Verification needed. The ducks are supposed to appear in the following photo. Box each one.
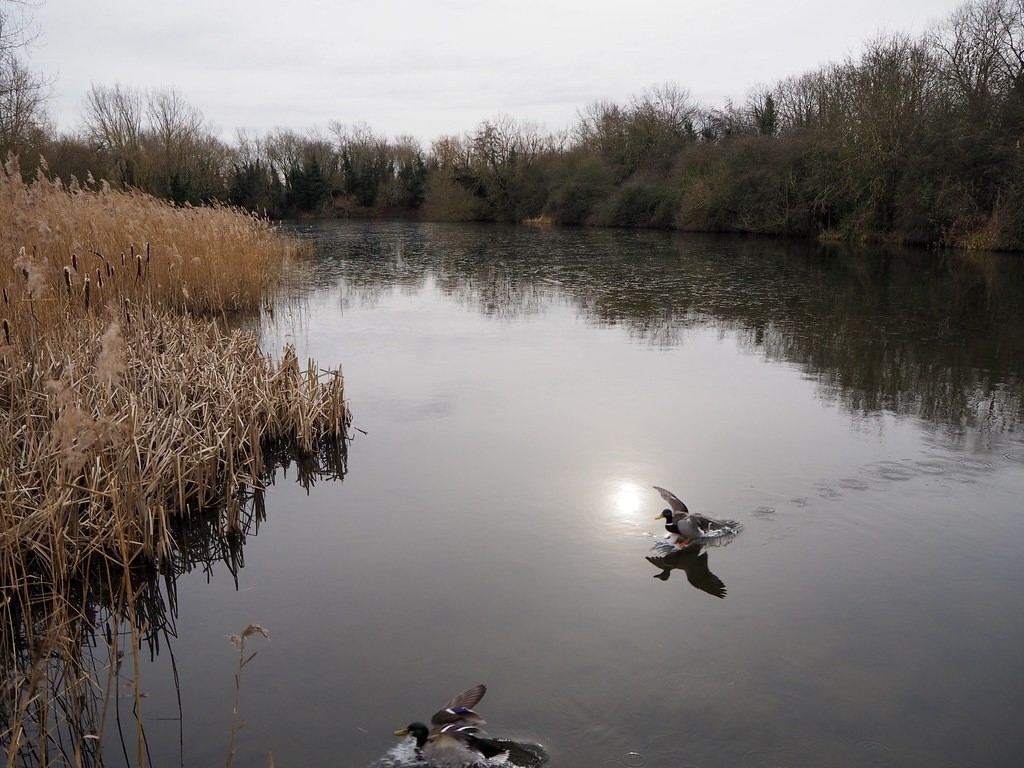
[653,486,724,548]
[393,684,549,768]
